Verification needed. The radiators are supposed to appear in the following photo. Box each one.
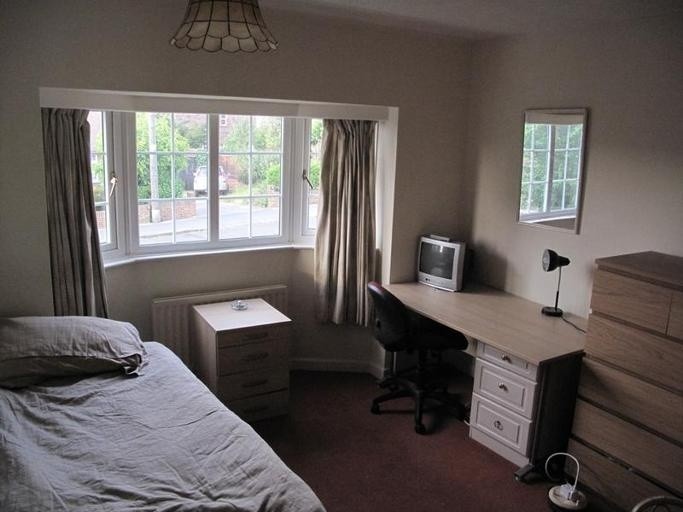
[151,284,289,367]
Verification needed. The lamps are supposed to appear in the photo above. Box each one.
[541,249,570,316]
[169,0,280,56]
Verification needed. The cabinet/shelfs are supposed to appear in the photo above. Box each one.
[561,250,683,512]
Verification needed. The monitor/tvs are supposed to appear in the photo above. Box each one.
[416,234,475,292]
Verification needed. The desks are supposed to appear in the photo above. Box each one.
[382,280,588,480]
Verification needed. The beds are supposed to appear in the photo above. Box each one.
[0,342,327,512]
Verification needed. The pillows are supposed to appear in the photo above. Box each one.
[0,315,146,390]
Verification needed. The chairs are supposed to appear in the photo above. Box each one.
[367,281,467,433]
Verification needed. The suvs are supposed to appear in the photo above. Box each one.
[193,164,230,197]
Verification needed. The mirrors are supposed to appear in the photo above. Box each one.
[512,106,588,236]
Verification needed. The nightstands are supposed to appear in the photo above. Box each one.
[193,297,295,426]
